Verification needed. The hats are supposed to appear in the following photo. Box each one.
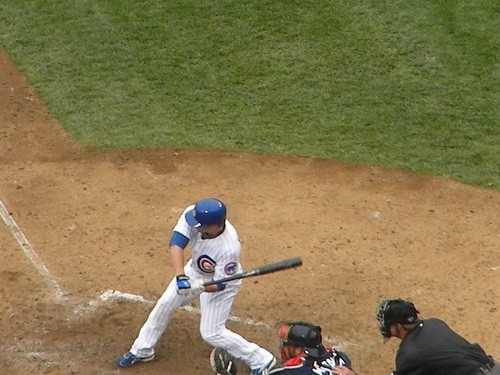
[185,199,227,228]
[377,299,418,344]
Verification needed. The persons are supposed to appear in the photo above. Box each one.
[331,299,500,374]
[115,198,277,375]
[266,322,351,374]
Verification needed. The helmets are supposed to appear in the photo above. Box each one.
[277,320,332,362]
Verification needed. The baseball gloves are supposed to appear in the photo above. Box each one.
[207,348,239,375]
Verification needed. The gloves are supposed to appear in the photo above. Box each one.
[191,280,204,296]
[177,275,191,296]
[210,347,238,375]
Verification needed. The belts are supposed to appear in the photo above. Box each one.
[470,355,495,375]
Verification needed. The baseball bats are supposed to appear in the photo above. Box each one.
[176,256,303,296]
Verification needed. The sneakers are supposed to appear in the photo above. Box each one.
[115,352,155,367]
[250,357,276,375]
